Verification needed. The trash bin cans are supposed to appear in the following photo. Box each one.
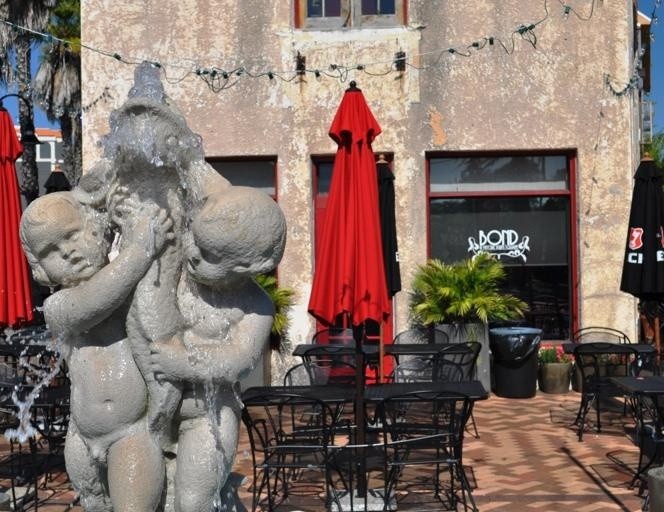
[489,326,543,398]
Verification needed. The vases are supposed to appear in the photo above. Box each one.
[538,363,570,394]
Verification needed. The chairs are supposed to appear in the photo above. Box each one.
[572,327,664,510]
[0,317,78,512]
[232,326,481,512]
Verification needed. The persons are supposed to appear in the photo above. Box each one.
[19,192,167,512]
[111,182,287,512]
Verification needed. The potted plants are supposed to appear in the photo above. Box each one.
[237,272,297,392]
[407,252,530,392]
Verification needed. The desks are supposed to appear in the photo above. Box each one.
[561,343,656,433]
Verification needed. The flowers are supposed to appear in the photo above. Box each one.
[535,338,576,375]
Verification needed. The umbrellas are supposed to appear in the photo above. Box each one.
[308,81,390,499]
[619,152,664,366]
[0,107,35,326]
